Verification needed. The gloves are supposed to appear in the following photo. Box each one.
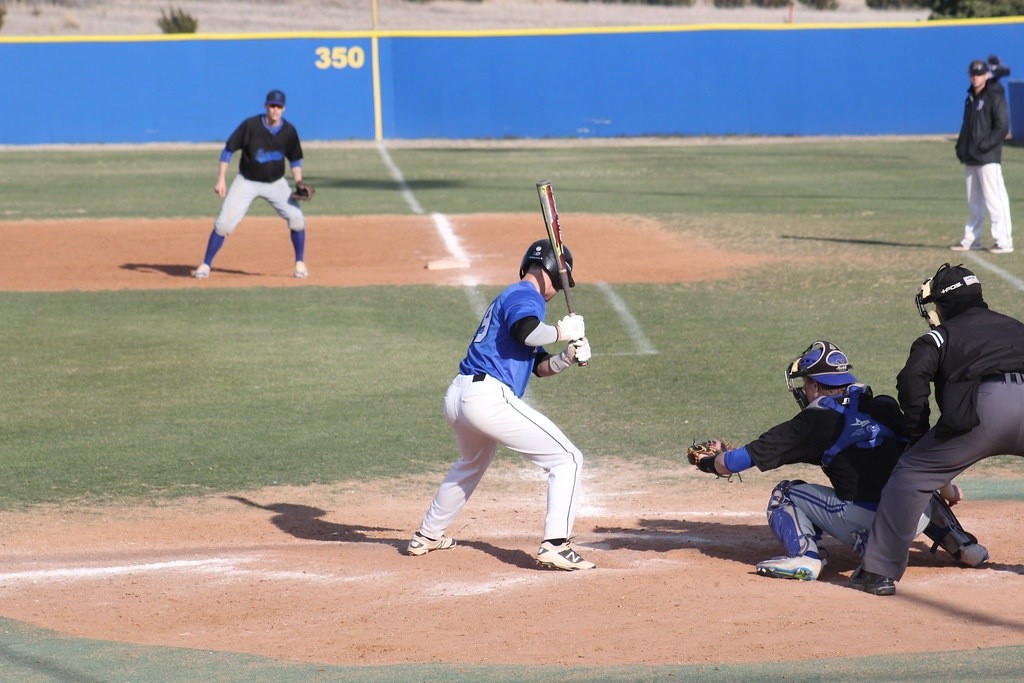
[557,314,585,341]
[565,337,592,363]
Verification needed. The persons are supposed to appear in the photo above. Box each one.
[195,89,314,279]
[849,264,1024,595]
[693,339,988,581]
[949,60,1015,254]
[407,238,597,570]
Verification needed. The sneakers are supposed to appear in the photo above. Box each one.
[406,531,457,556]
[960,543,988,568]
[534,536,596,570]
[756,554,827,581]
[848,572,895,595]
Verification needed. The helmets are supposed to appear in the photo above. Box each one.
[799,347,857,386]
[520,239,575,287]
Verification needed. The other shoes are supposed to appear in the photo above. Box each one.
[988,245,1014,253]
[195,263,210,278]
[951,242,982,250]
[295,261,307,278]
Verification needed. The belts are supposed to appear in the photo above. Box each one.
[472,374,485,382]
[853,499,879,512]
[981,374,1024,382]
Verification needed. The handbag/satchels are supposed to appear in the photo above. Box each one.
[935,378,981,440]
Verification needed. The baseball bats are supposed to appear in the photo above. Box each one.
[536,179,590,366]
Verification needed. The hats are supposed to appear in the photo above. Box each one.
[969,61,989,74]
[267,90,285,107]
[931,266,982,302]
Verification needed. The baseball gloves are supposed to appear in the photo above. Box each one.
[688,437,738,475]
[290,182,316,201]
